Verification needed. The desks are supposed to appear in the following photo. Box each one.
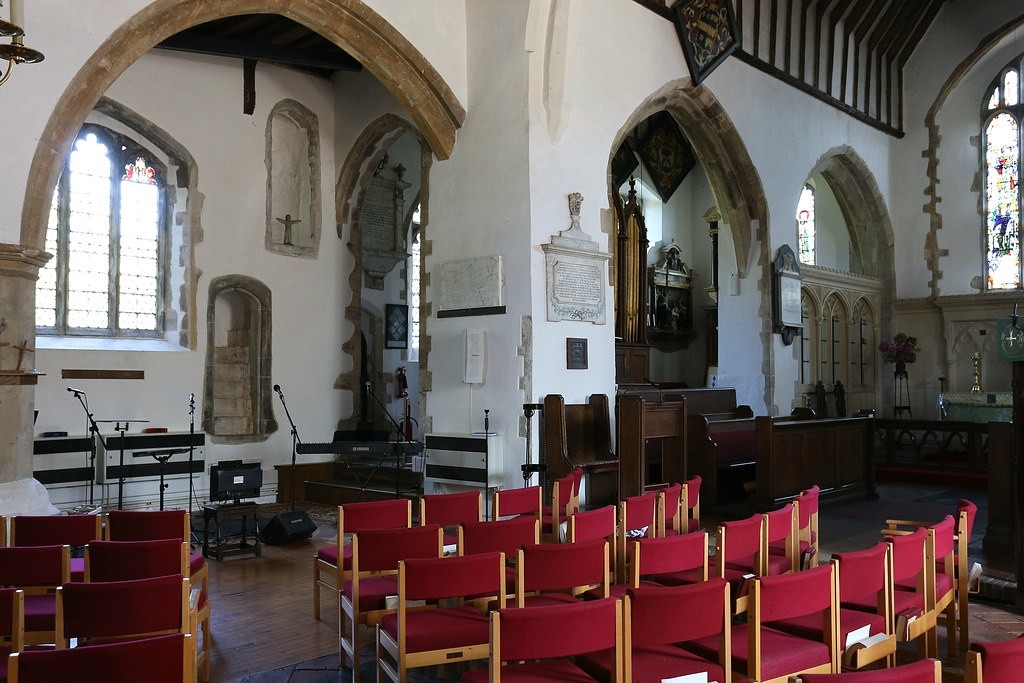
[939,392,1013,407]
[201,502,263,562]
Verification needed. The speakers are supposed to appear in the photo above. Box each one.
[261,511,317,545]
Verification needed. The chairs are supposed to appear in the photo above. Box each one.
[0,510,210,683]
[313,465,1024,683]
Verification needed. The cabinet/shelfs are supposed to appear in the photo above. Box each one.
[424,433,503,489]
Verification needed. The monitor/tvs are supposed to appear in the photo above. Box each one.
[210,463,263,508]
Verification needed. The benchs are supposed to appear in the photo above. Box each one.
[689,406,816,511]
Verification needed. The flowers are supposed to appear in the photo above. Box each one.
[878,332,919,365]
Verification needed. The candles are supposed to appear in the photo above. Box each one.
[9,0,25,35]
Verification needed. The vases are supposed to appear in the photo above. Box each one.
[895,362,906,374]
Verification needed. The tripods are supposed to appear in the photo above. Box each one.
[189,402,215,544]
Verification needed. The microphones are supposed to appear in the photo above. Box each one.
[366,381,371,397]
[643,377,662,402]
[189,392,194,402]
[66,387,85,394]
[273,384,284,396]
